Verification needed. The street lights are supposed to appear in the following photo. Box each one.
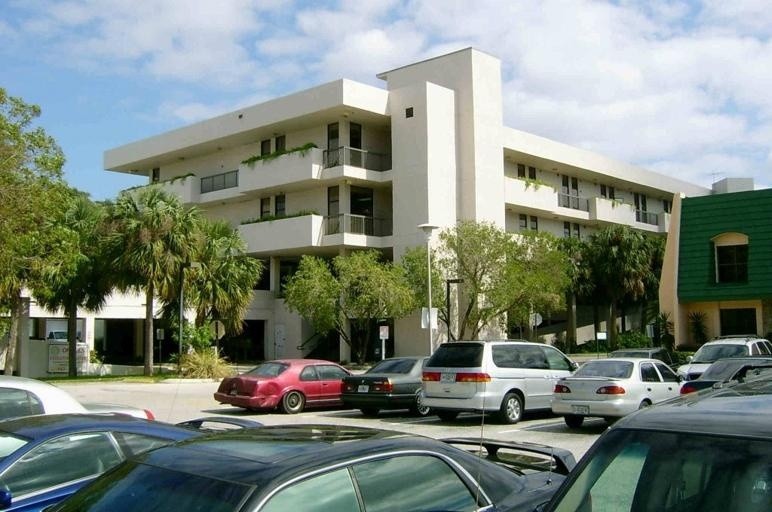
[418,223,440,356]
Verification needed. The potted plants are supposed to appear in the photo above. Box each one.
[656,313,674,349]
